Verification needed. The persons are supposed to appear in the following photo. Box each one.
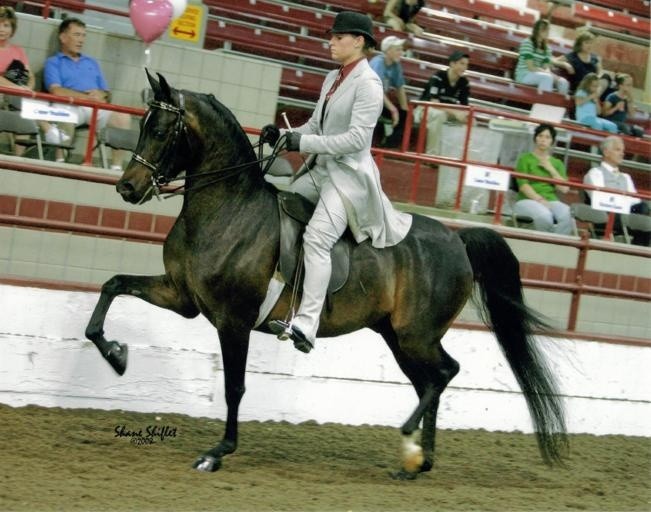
[603,73,643,137]
[43,18,132,171]
[383,0,425,37]
[512,124,579,236]
[582,135,651,247]
[515,19,569,95]
[259,11,413,353]
[0,7,70,156]
[553,32,612,97]
[412,50,470,124]
[368,35,409,150]
[574,72,618,134]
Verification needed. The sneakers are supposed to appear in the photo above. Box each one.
[44,123,60,147]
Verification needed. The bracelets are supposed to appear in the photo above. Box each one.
[537,198,544,202]
[391,108,398,114]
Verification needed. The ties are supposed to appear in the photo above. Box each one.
[324,67,343,101]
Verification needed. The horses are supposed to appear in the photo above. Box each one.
[85,66,575,483]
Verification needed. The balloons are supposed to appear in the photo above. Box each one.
[128,0,173,44]
[172,0,187,20]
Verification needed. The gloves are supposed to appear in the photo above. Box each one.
[285,130,301,151]
[260,124,280,147]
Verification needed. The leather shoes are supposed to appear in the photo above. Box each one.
[267,319,311,354]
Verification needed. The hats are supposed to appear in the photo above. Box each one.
[325,10,377,47]
[380,35,406,51]
[448,50,470,61]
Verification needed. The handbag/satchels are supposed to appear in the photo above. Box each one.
[2,59,29,86]
